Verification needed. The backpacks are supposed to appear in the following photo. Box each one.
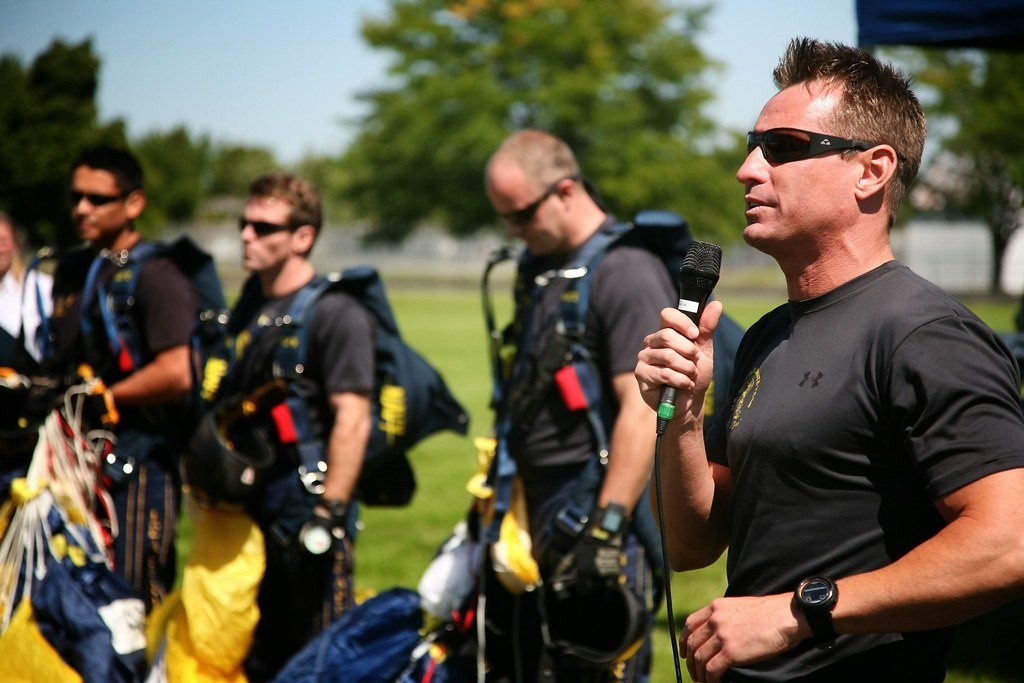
[110,237,228,350]
[286,266,469,455]
[569,212,755,462]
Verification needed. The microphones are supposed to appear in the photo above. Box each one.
[656,241,722,435]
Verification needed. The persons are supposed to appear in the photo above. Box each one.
[481,37,1024,683]
[1,143,380,683]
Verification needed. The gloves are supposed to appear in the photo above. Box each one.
[296,497,355,575]
[552,502,630,592]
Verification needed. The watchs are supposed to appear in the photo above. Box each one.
[794,575,838,651]
[319,497,347,517]
[590,502,634,535]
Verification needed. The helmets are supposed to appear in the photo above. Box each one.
[547,546,655,665]
[185,410,273,515]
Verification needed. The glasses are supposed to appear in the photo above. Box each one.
[505,176,578,225]
[747,128,906,163]
[238,216,298,237]
[69,192,129,205]
